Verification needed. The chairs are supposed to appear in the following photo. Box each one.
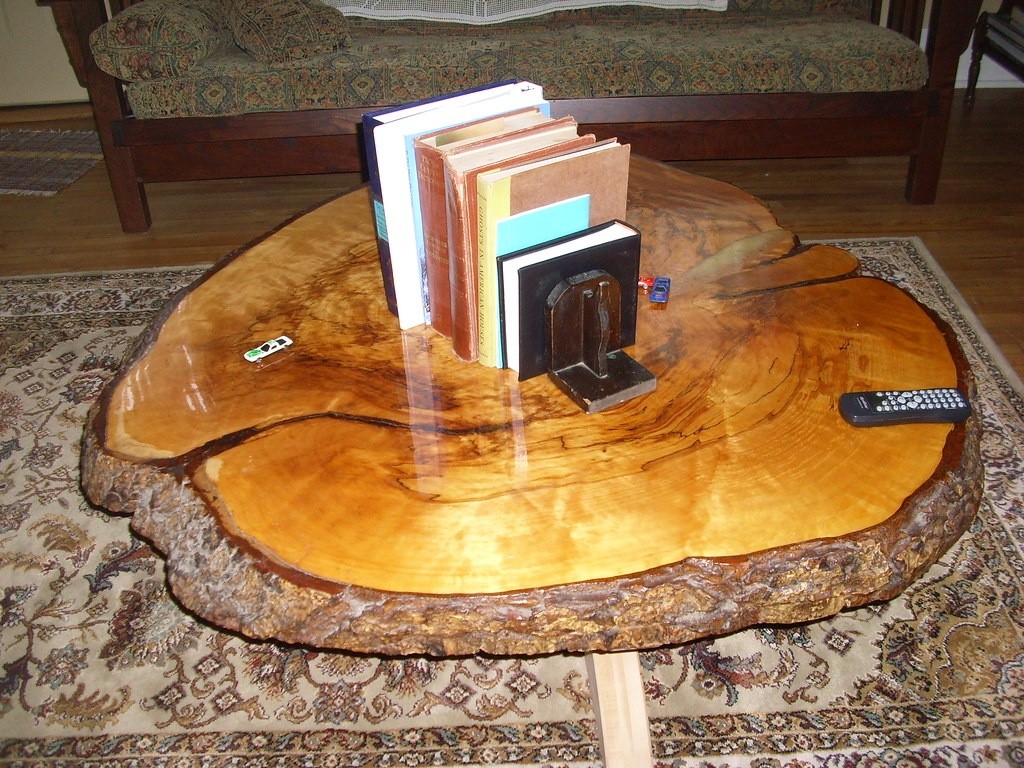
[962,0,1024,116]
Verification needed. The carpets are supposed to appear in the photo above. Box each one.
[0,126,104,198]
[0,235,1024,767]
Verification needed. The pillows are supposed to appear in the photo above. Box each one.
[220,0,354,64]
[88,0,237,84]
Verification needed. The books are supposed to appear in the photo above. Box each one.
[360,78,642,381]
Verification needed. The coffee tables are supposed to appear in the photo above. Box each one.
[81,149,986,658]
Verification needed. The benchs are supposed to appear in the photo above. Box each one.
[36,0,982,236]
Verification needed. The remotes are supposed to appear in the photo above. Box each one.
[837,388,972,427]
